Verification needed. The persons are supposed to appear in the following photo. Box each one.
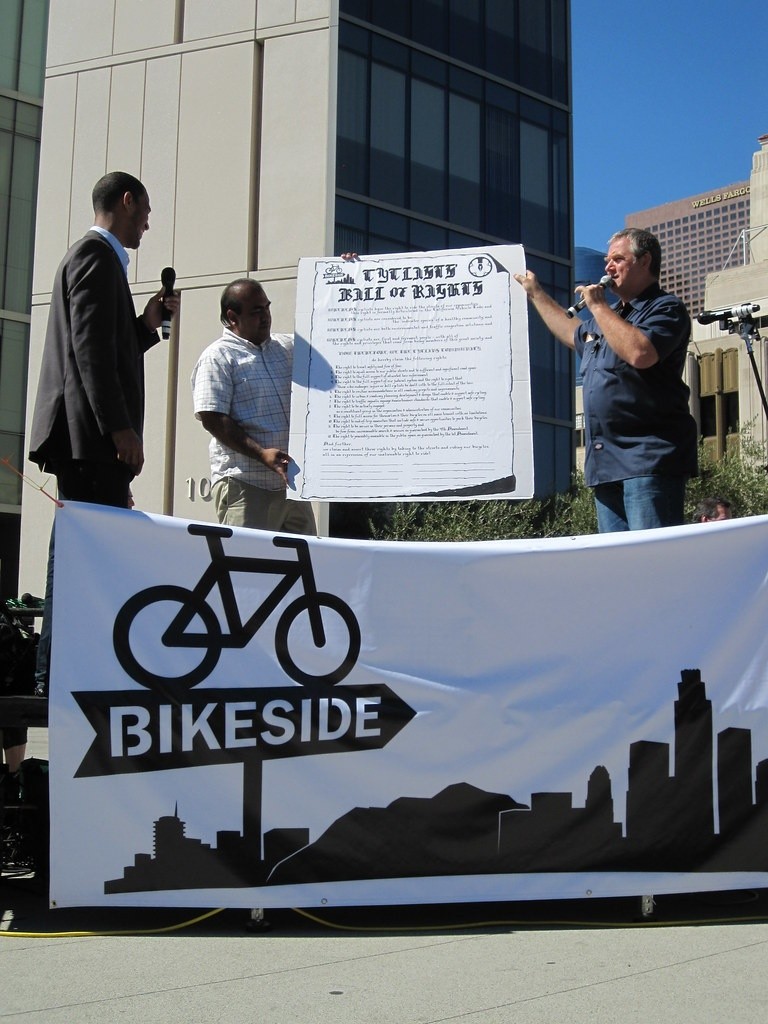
[691,494,733,522]
[29,169,178,695]
[193,279,321,535]
[515,225,698,532]
[0,606,33,793]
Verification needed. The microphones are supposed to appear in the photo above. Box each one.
[161,267,176,339]
[697,304,760,325]
[565,275,614,318]
